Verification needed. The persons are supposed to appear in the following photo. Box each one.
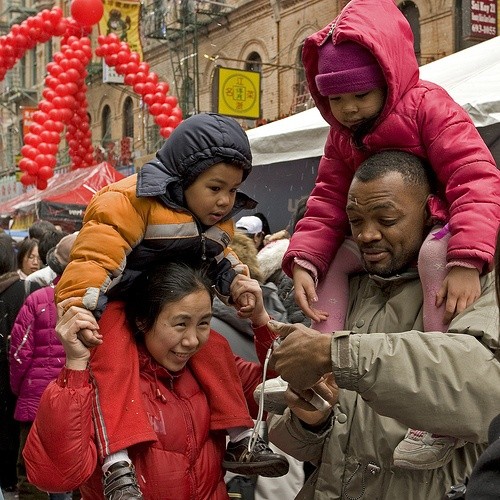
[464,437,500,500]
[56,114,288,500]
[15,195,312,361]
[266,154,499,500]
[252,0,500,469]
[10,230,81,500]
[0,232,26,491]
[21,258,279,500]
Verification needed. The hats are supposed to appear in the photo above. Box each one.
[315,34,386,95]
[46,231,80,274]
[236,216,264,234]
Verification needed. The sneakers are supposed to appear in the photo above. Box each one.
[253,376,289,415]
[104,461,144,500]
[393,428,468,471]
[222,434,289,477]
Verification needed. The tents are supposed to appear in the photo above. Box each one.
[0,161,127,232]
[233,36,499,173]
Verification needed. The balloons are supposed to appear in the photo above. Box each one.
[0,1,186,190]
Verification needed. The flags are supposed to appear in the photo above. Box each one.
[97,2,144,84]
